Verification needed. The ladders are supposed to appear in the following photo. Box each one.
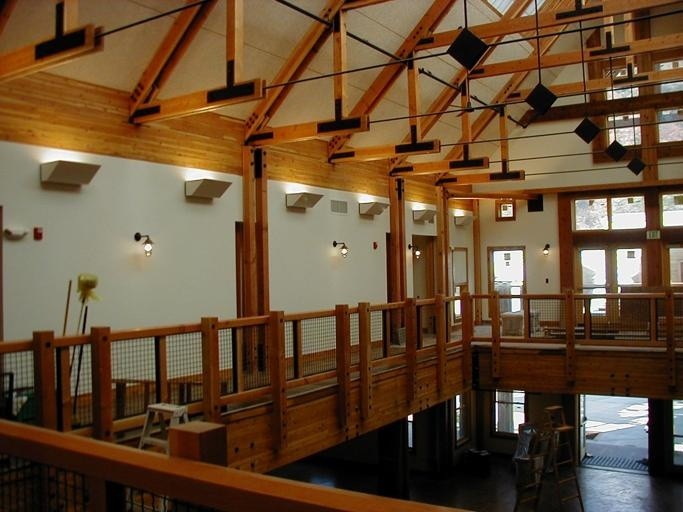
[123,403,190,512]
[513,405,584,512]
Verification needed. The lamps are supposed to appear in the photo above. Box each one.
[408,243,422,261]
[358,201,389,218]
[542,243,551,256]
[333,241,351,258]
[132,231,155,257]
[284,192,323,211]
[413,207,476,226]
[38,157,102,190]
[185,178,232,202]
